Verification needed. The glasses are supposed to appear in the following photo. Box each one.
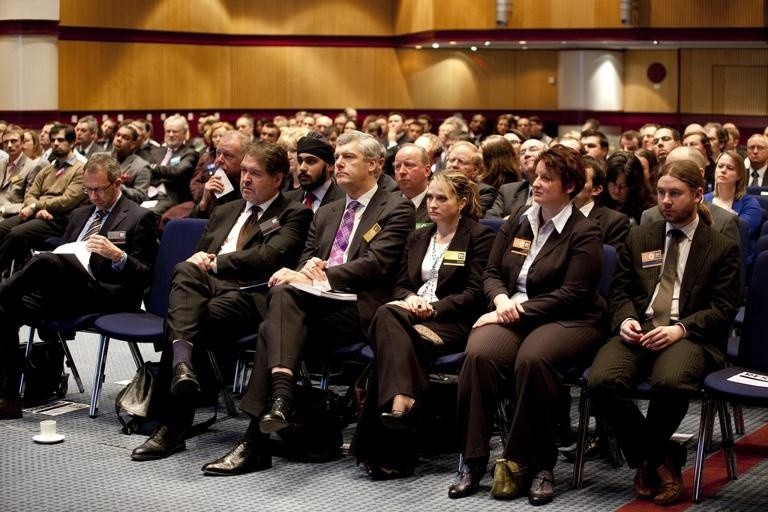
[82,183,112,194]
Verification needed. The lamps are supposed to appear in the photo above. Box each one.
[617,2,641,26]
[493,0,514,27]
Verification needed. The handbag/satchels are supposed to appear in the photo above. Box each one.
[605,429,625,467]
[350,385,420,472]
[265,382,343,463]
[19,342,69,407]
[425,370,463,454]
[490,457,530,500]
[115,362,219,440]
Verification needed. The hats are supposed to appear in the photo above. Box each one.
[296,132,334,164]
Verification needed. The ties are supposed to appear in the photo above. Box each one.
[81,211,106,241]
[236,207,263,251]
[652,231,681,327]
[327,202,360,267]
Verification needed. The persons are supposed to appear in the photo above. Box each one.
[2,107,767,508]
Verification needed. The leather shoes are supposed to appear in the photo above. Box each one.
[259,398,295,434]
[380,399,419,428]
[202,439,272,476]
[449,464,487,498]
[564,433,603,460]
[171,361,201,397]
[529,466,554,506]
[131,426,187,461]
[633,440,688,505]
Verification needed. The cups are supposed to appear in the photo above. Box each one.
[40,419,57,439]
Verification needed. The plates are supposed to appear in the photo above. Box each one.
[32,434,66,444]
